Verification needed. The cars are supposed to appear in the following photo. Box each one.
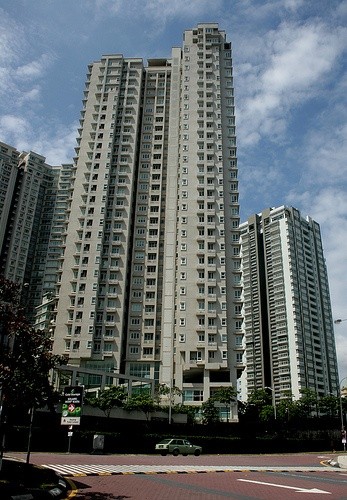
[155,438,203,456]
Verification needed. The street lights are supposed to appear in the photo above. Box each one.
[263,386,277,421]
